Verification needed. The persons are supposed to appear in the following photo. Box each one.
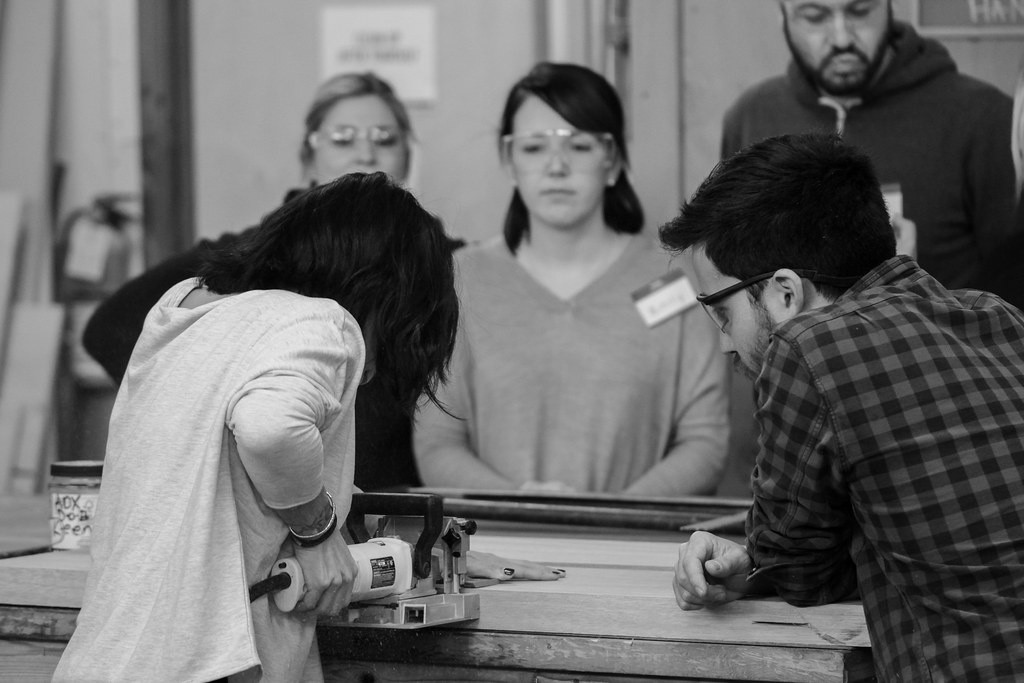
[46,170,568,683]
[661,134,1024,683]
[719,1,1024,312]
[81,70,425,491]
[407,62,736,540]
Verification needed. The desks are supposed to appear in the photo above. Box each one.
[0,519,882,683]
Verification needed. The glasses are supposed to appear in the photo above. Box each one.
[695,269,817,334]
[502,129,616,171]
[308,128,403,152]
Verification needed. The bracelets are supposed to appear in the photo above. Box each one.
[291,515,337,548]
[286,491,336,538]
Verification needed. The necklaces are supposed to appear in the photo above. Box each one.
[526,224,610,285]
